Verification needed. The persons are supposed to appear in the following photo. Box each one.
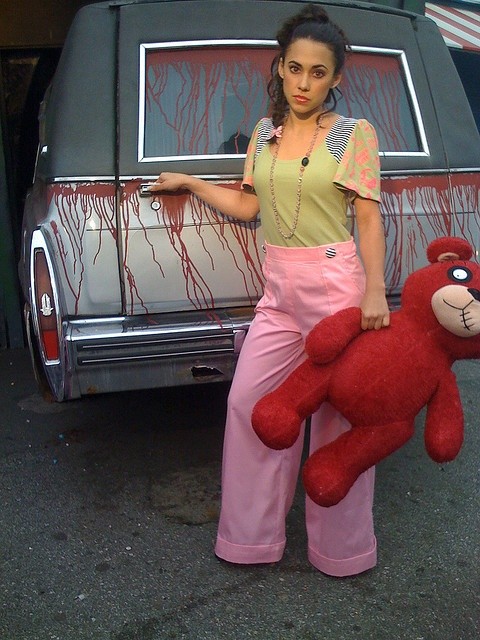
[148,3,389,578]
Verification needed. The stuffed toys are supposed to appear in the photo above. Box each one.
[248,235,480,508]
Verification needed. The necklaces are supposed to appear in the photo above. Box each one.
[268,107,326,238]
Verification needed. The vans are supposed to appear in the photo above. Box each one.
[18,0,479,404]
